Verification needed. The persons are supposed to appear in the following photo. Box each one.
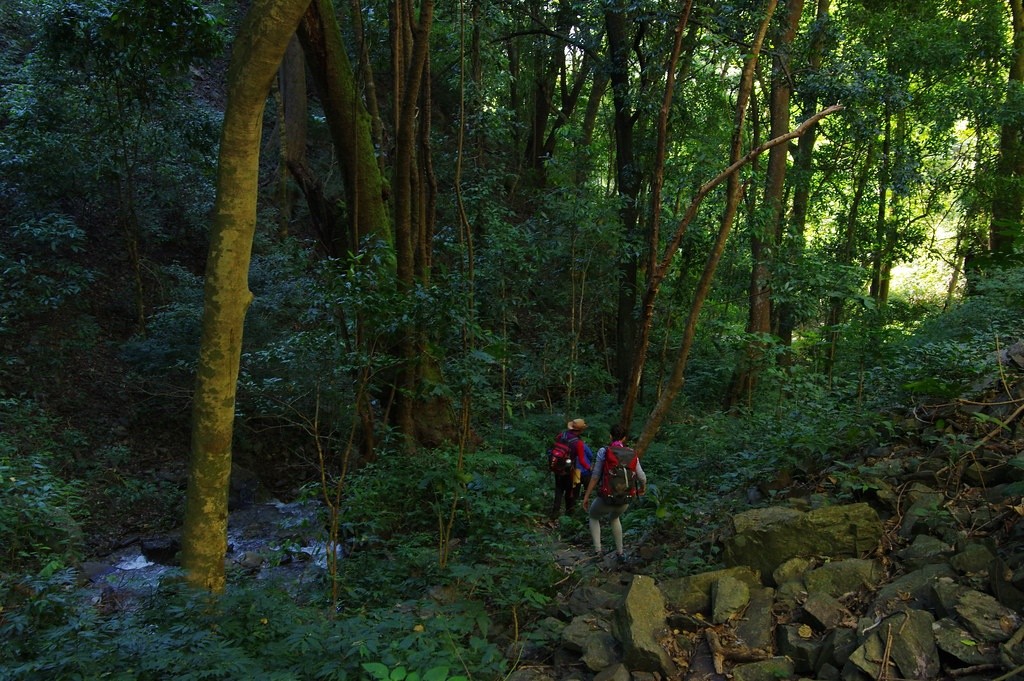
[573,435,594,505]
[552,419,592,516]
[582,422,648,567]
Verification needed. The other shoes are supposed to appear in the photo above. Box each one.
[596,551,627,563]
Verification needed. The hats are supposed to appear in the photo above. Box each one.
[568,419,588,431]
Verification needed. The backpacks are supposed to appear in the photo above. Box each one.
[549,431,581,473]
[596,444,638,505]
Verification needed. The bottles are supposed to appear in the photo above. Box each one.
[574,467,580,483]
